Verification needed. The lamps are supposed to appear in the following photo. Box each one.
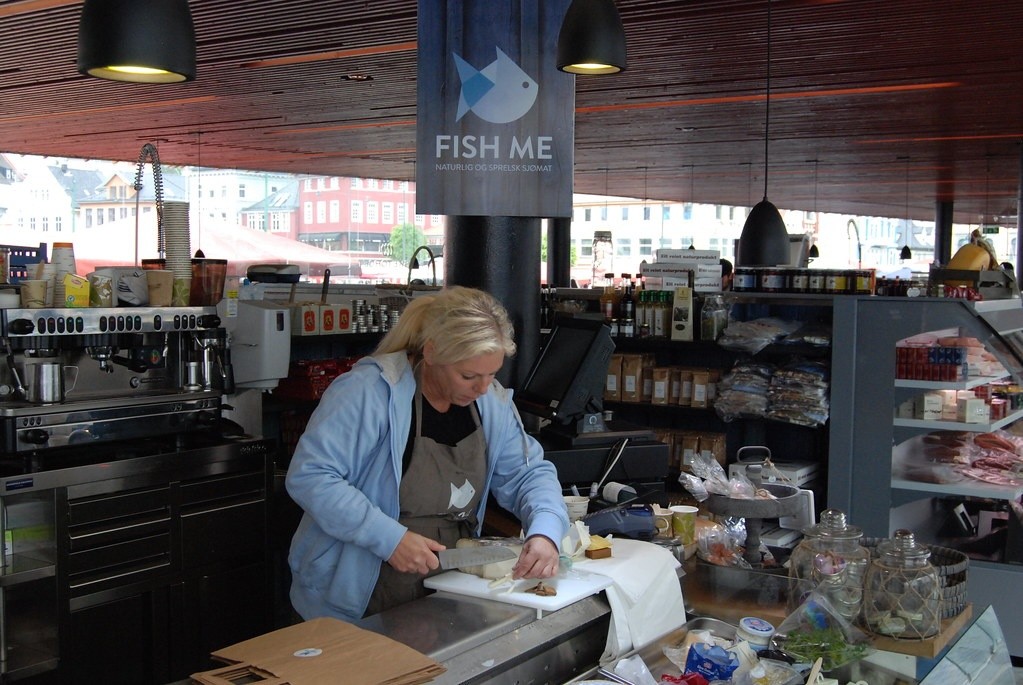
[736,0,792,267]
[688,166,695,250]
[555,0,628,76]
[808,162,819,258]
[639,167,646,272]
[76,0,195,84]
[900,160,911,260]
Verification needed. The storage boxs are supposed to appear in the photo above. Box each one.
[979,384,993,401]
[992,398,1011,418]
[987,401,1005,420]
[728,453,822,487]
[895,345,968,382]
[934,389,956,404]
[914,393,943,420]
[956,390,986,423]
[942,404,957,419]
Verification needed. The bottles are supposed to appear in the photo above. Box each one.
[541,282,557,328]
[601,273,728,342]
[784,509,869,631]
[863,531,942,639]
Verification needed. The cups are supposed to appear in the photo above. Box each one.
[602,482,636,503]
[671,506,699,544]
[146,201,192,307]
[18,243,77,308]
[654,508,675,539]
[563,496,591,522]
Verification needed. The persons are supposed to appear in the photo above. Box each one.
[285,286,568,627]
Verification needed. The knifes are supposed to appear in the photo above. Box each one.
[432,546,517,570]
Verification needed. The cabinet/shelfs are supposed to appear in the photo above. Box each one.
[542,288,1023,659]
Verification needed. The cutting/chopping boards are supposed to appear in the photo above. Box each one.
[423,567,614,611]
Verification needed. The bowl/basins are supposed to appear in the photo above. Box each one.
[142,259,227,307]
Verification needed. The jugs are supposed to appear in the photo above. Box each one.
[8,356,79,404]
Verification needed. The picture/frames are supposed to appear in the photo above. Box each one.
[952,503,977,537]
